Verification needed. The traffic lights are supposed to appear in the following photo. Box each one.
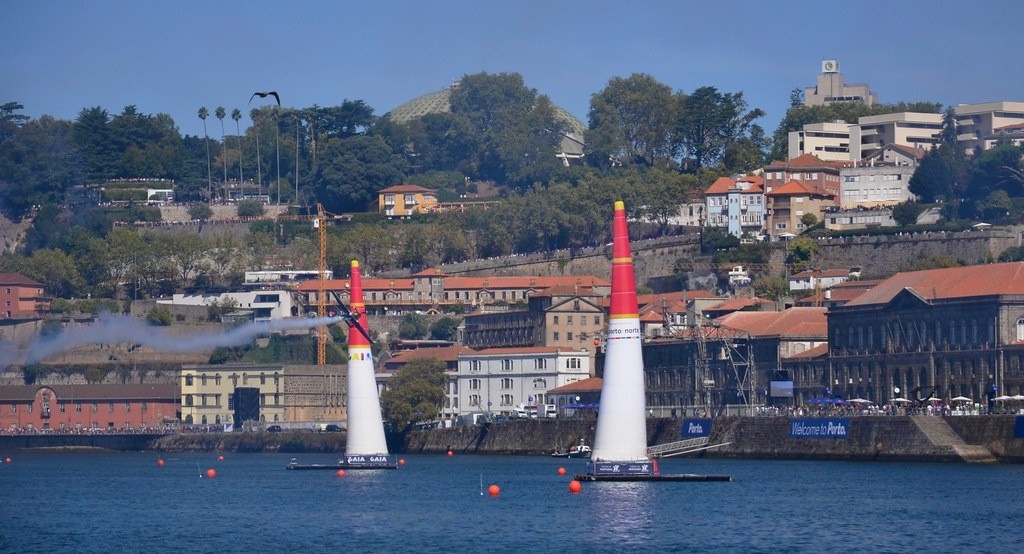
[594,337,600,346]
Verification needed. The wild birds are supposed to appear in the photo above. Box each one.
[248,90,280,108]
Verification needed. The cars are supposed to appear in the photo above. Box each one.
[326,424,341,433]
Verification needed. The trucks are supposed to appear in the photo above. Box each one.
[512,410,528,419]
[536,405,558,419]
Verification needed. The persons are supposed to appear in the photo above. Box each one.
[755,402,989,415]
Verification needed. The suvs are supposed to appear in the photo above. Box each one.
[266,425,281,432]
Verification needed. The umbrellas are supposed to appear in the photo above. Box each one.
[991,393,1024,407]
[951,395,973,403]
[916,396,942,401]
[806,396,872,402]
[889,397,912,402]
